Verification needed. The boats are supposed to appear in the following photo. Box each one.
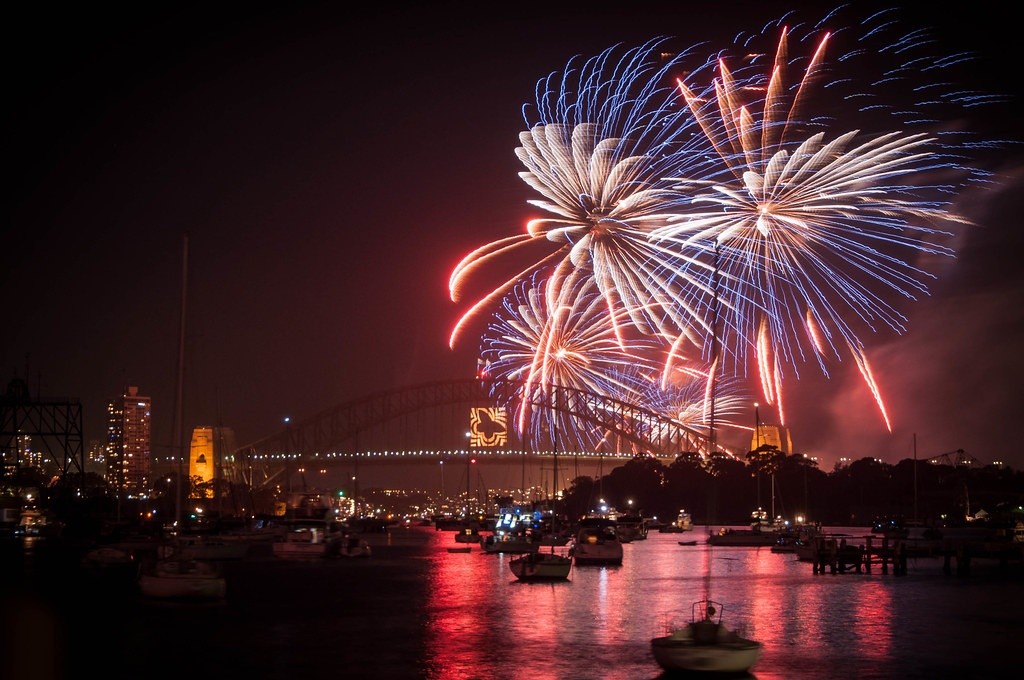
[650,530,760,673]
[433,433,693,553]
[163,418,400,543]
[509,425,572,579]
[572,456,623,564]
[705,407,945,561]
